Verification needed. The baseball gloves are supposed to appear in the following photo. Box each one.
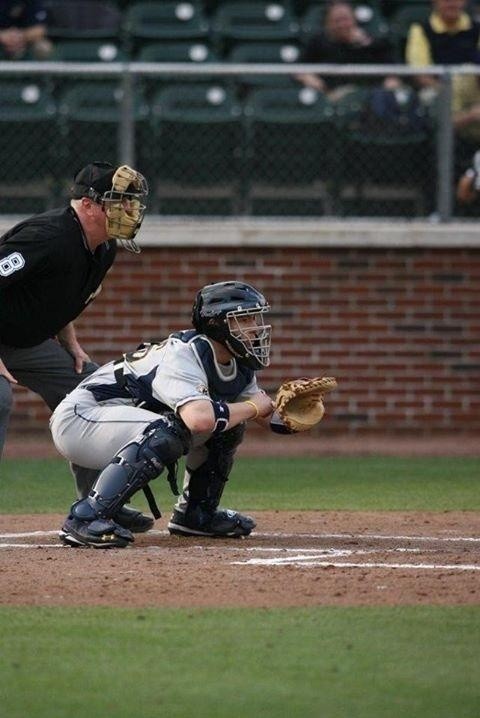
[271,377,337,432]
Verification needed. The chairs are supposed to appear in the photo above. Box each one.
[0,2,478,218]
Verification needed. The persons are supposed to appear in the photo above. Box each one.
[0,0,53,65]
[406,0,480,216]
[0,162,155,533]
[289,1,406,106]
[48,280,337,550]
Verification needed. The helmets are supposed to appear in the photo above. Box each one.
[192,280,270,370]
[75,161,146,199]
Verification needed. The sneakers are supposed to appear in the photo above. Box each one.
[167,504,257,538]
[58,506,155,548]
